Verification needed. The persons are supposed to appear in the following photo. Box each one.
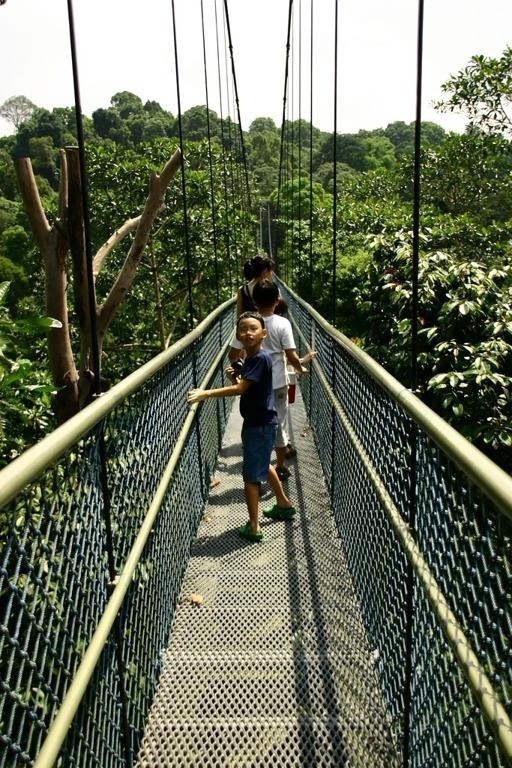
[236,254,275,314]
[274,295,318,459]
[187,310,297,540]
[229,278,309,482]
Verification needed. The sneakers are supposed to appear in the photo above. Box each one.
[274,467,291,480]
[286,449,297,457]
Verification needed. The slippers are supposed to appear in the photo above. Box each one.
[263,505,296,517]
[240,522,266,540]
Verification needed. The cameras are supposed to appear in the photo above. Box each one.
[232,358,246,377]
